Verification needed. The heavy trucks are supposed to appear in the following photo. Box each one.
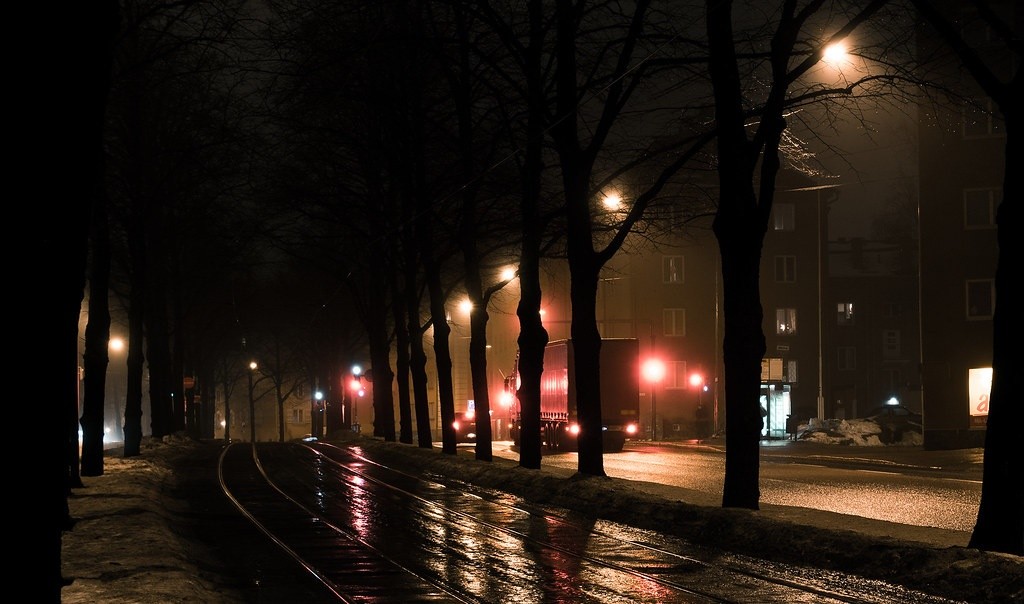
[503,336,640,452]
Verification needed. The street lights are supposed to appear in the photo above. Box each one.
[349,364,365,423]
[600,189,627,339]
[690,362,704,444]
[641,334,662,441]
[490,262,520,440]
[816,32,849,421]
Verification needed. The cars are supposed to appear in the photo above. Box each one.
[840,396,922,443]
[454,411,477,443]
